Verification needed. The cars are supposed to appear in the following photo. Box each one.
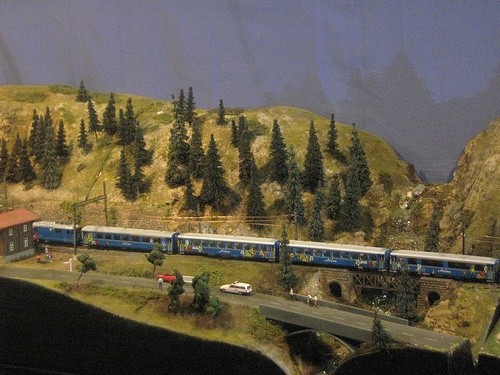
[154,273,177,283]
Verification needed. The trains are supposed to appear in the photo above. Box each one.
[33,221,500,284]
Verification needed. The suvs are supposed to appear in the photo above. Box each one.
[220,281,252,295]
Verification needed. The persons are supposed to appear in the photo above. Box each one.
[307,293,312,304]
[158,276,163,288]
[289,287,293,300]
[313,295,318,307]
